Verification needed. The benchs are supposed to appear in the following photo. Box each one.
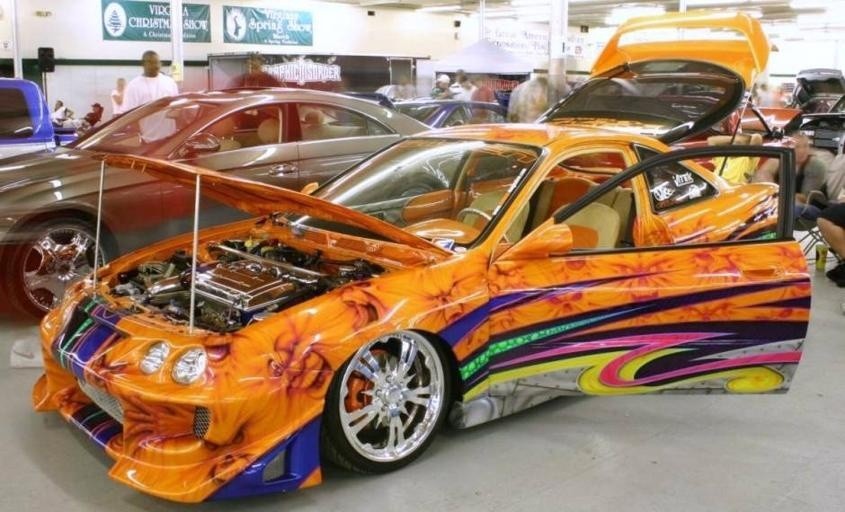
[530,177,633,248]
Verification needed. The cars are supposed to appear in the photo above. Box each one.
[31,122,812,506]
[393,100,520,128]
[536,8,845,288]
[0,86,431,312]
[342,92,396,110]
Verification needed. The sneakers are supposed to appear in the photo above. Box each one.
[826,262,844,287]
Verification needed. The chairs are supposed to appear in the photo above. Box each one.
[77,107,103,131]
[206,109,242,152]
[795,155,845,257]
[464,191,530,242]
[553,202,619,250]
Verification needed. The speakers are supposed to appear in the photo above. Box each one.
[39,47,55,72]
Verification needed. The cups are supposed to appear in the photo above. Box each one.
[815,244,828,269]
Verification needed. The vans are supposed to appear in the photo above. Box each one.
[0,76,79,159]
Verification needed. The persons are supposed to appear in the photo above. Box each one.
[110,78,127,119]
[224,53,288,130]
[815,201,845,288]
[373,69,500,125]
[50,100,73,128]
[753,132,830,232]
[76,103,104,137]
[505,59,571,125]
[119,50,181,147]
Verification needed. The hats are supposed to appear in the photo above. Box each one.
[438,74,450,83]
